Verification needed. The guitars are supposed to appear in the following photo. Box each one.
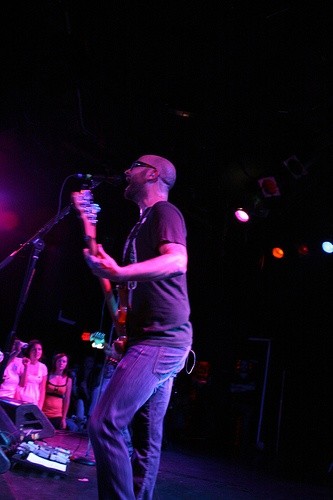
[72,190,132,355]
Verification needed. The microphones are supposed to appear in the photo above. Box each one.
[76,173,122,183]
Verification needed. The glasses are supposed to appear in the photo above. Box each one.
[131,160,156,170]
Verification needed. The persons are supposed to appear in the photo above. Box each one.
[1,331,26,402]
[81,156,193,500]
[43,353,73,431]
[16,339,49,412]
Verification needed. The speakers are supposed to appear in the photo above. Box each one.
[0,397,55,473]
